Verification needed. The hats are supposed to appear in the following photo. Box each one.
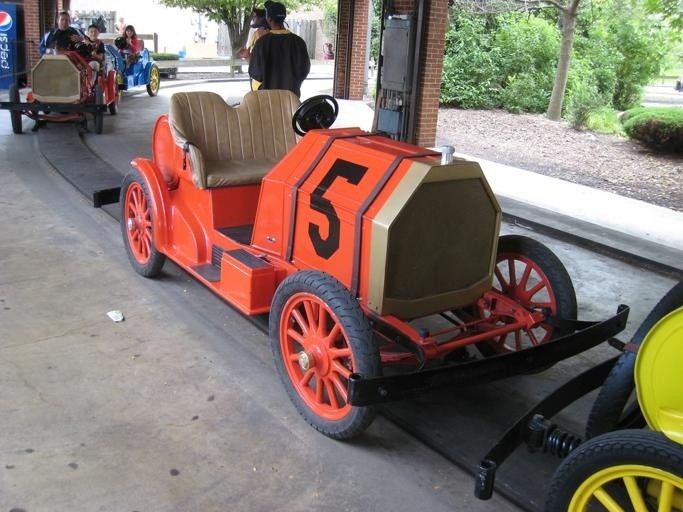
[264,0,287,23]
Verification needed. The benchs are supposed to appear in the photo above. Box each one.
[169,88,305,190]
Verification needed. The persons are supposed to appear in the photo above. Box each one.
[37,11,91,60]
[248,1,310,100]
[80,25,104,90]
[235,8,270,93]
[116,25,140,70]
